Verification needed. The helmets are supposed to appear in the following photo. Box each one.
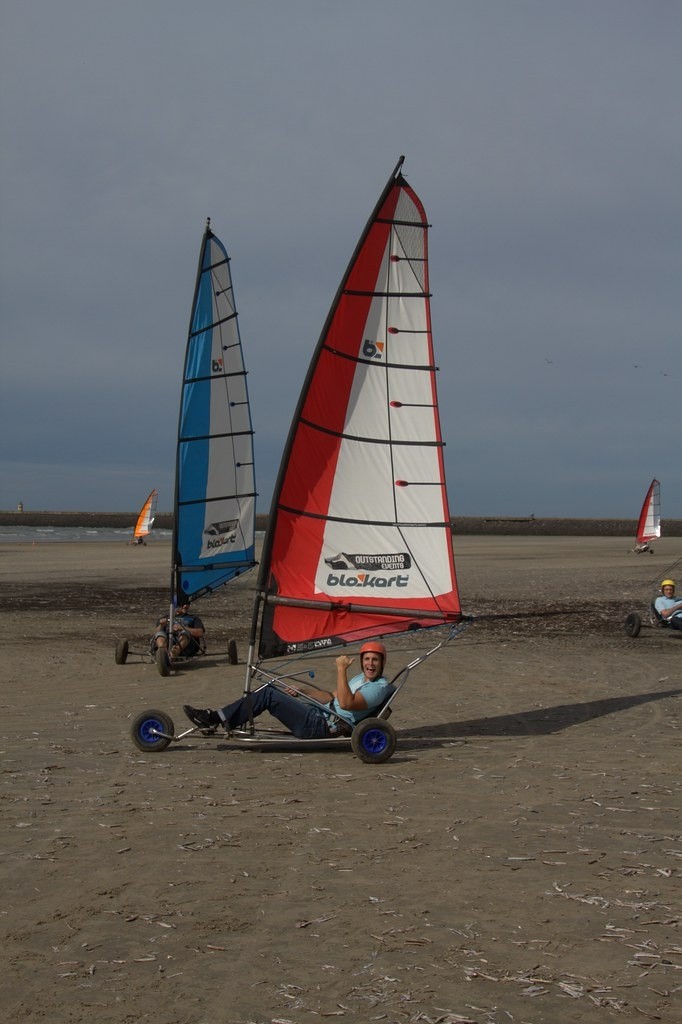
[661,579,676,595]
[360,641,386,664]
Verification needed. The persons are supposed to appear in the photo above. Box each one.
[151,600,205,657]
[654,579,682,630]
[183,642,389,740]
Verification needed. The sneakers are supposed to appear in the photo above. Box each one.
[183,705,218,735]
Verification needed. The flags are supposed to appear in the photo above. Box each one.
[637,479,662,542]
[178,229,258,606]
[134,489,158,537]
[258,173,462,658]
